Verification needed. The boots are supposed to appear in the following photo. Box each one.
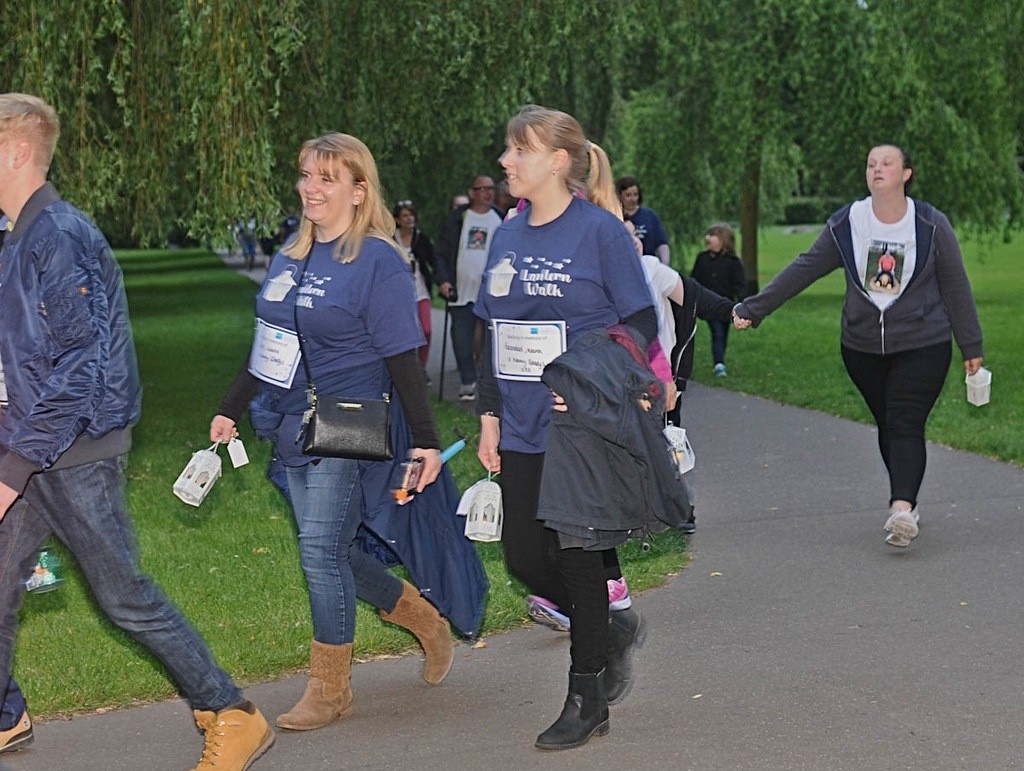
[533,669,611,750]
[601,606,648,705]
[274,637,354,730]
[380,578,454,684]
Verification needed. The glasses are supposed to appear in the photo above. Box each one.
[471,186,496,192]
[395,200,413,210]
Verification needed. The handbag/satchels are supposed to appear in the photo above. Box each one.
[294,396,393,460]
[421,252,448,285]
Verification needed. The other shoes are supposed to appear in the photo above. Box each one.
[0,708,34,753]
[423,369,433,388]
[683,506,697,534]
[714,364,726,378]
[458,387,476,402]
[883,502,921,548]
[193,700,276,770]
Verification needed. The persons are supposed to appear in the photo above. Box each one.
[492,181,517,209]
[615,175,669,266]
[476,107,659,752]
[689,224,747,380]
[0,92,274,771]
[623,219,755,464]
[384,200,434,378]
[732,143,986,548]
[434,176,509,402]
[207,133,454,733]
[225,205,299,275]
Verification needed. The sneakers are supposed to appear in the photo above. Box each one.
[605,577,632,610]
[525,593,571,632]
